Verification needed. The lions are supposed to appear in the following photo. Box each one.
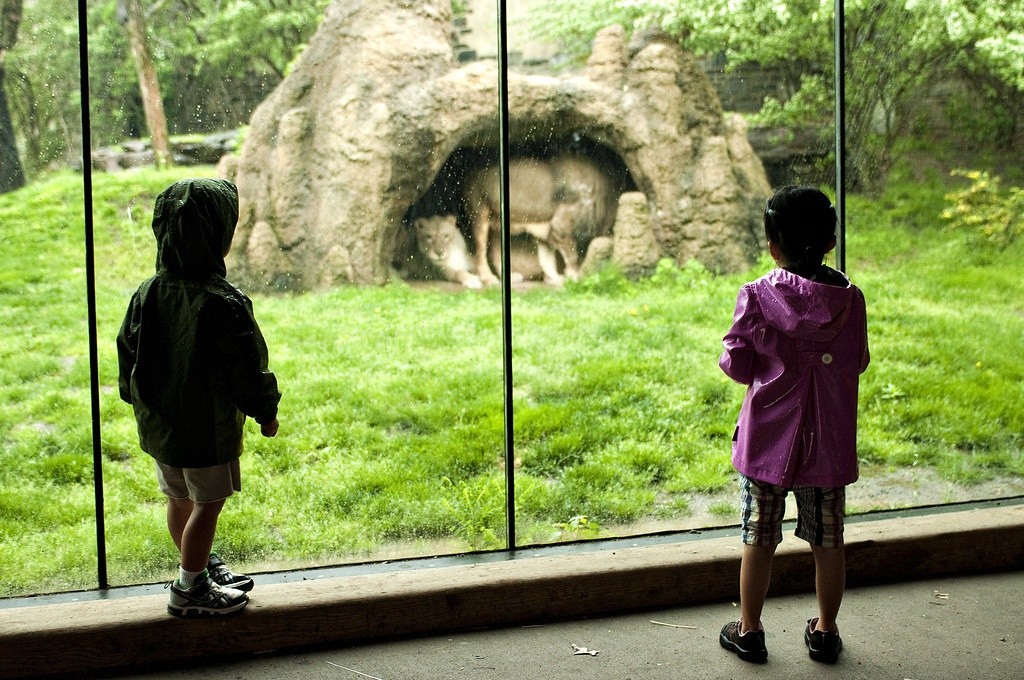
[394,151,619,291]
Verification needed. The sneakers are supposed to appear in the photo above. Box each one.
[804,618,842,660]
[720,618,768,663]
[207,550,254,593]
[164,571,249,617]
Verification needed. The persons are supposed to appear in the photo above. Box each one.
[719,185,872,665]
[116,177,281,618]
